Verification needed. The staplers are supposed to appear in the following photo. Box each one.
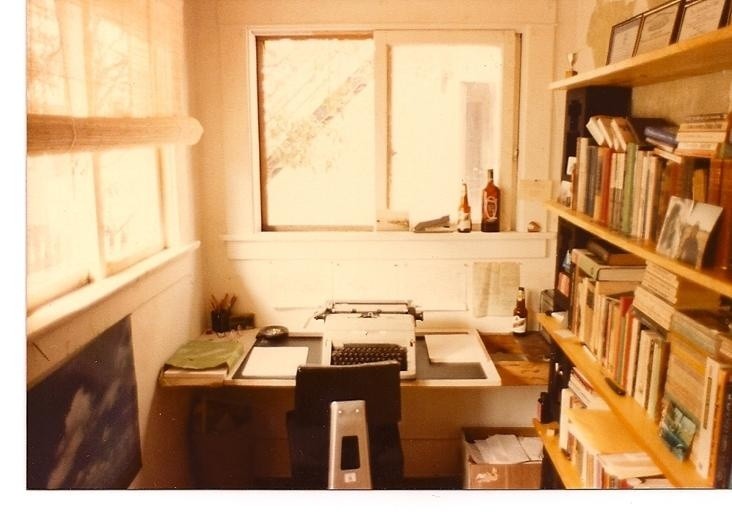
[412,216,455,233]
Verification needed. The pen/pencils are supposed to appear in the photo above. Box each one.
[208,293,237,313]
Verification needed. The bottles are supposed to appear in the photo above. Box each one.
[457,183,472,232]
[512,287,528,335]
[481,169,500,232]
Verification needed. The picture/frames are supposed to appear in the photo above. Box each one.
[606,0,732,66]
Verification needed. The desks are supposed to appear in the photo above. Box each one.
[155,320,550,439]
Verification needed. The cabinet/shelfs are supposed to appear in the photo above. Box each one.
[533,25,732,489]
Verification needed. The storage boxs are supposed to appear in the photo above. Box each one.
[460,426,544,490]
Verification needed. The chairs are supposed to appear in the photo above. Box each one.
[285,360,404,489]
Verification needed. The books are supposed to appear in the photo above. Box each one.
[557,113,732,271]
[422,333,491,365]
[557,238,732,490]
